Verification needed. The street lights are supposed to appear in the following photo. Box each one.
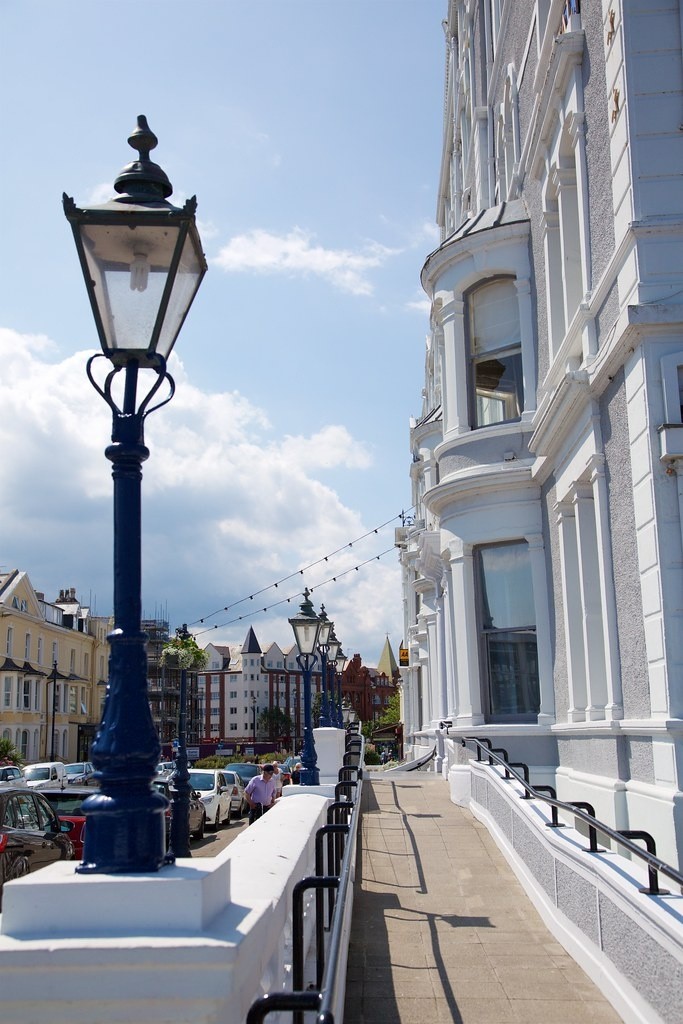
[292,689,298,756]
[288,587,325,786]
[317,604,334,727]
[334,647,348,729]
[166,623,198,858]
[61,114,208,874]
[372,684,376,724]
[252,696,257,737]
[320,631,342,729]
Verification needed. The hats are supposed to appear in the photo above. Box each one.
[261,764,274,772]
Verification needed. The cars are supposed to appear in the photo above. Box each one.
[21,762,68,788]
[0,766,27,788]
[381,747,395,756]
[224,756,301,788]
[156,759,193,775]
[0,788,75,913]
[152,776,207,844]
[38,786,101,860]
[210,770,250,819]
[64,762,98,786]
[163,768,232,832]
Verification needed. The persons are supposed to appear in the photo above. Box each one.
[271,761,284,802]
[289,762,302,785]
[380,749,387,765]
[387,750,393,762]
[244,764,276,826]
